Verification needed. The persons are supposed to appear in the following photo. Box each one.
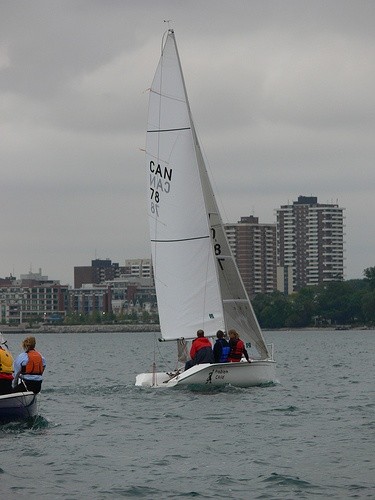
[0,335,46,395]
[212,330,230,363]
[227,329,251,363]
[184,329,213,371]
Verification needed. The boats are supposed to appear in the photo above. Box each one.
[0,388,39,423]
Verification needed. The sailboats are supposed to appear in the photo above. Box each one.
[135,24,277,391]
